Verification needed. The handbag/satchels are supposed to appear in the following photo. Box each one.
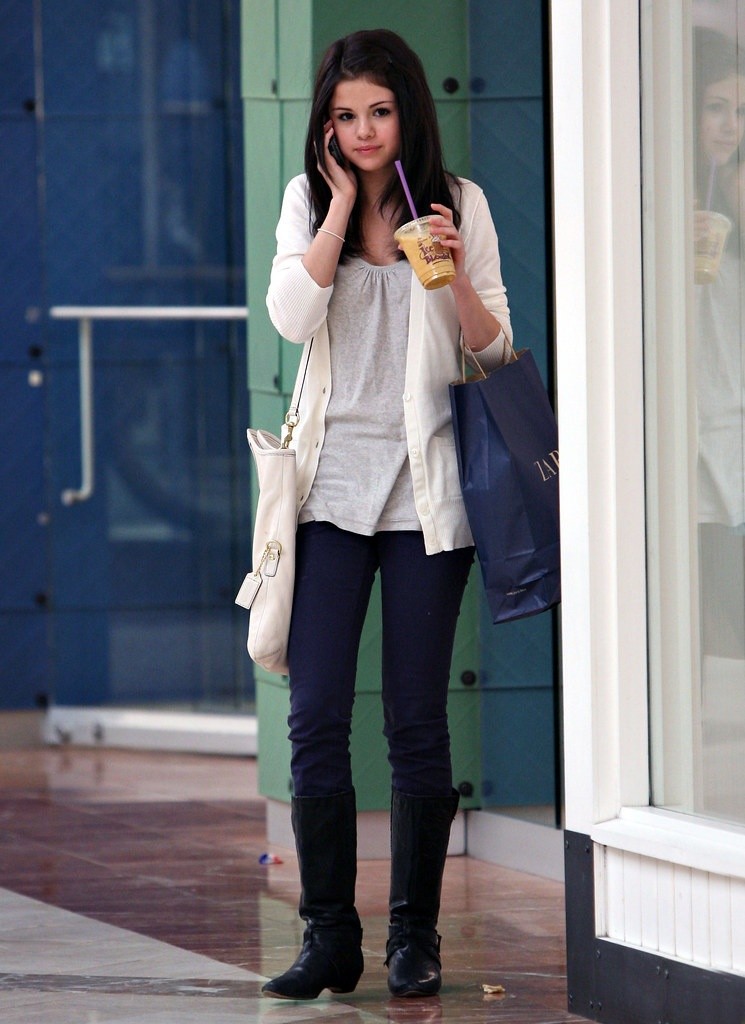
[447,318,561,626]
[234,428,298,677]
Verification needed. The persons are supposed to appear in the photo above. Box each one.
[671,19,745,666]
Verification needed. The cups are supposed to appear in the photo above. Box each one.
[695,210,735,282]
[394,213,458,291]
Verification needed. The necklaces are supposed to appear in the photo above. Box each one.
[261,29,522,1006]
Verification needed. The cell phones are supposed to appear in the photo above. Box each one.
[328,134,345,170]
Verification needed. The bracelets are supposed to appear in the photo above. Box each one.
[316,227,346,244]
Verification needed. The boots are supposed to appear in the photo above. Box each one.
[382,786,461,997]
[260,785,364,1001]
